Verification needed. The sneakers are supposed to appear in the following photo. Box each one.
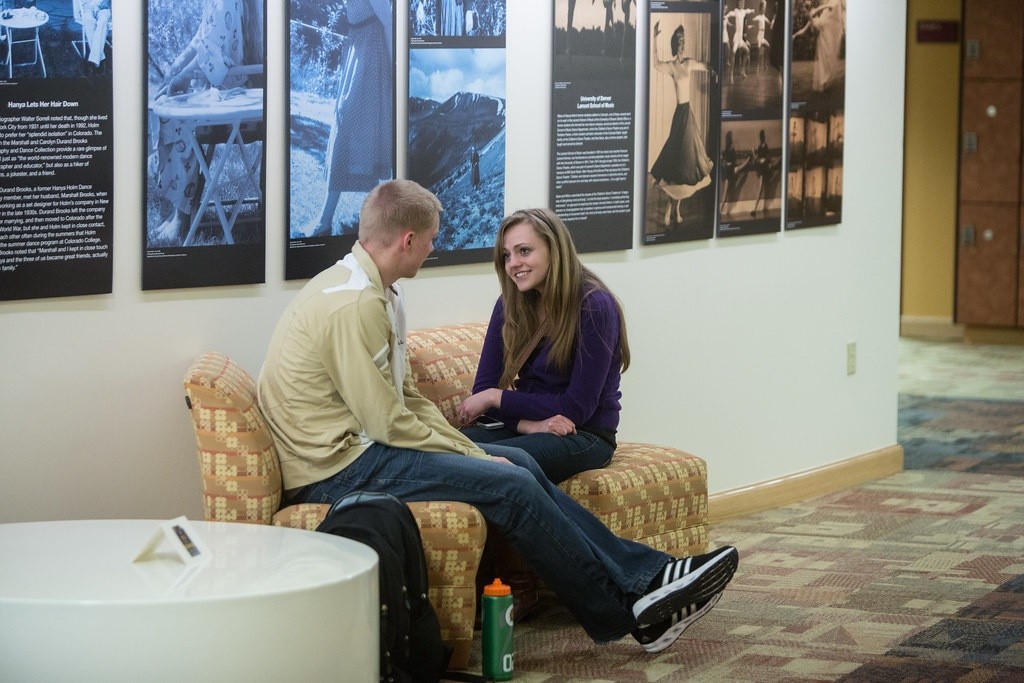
[629,545,739,624]
[631,591,723,653]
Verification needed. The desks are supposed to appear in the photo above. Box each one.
[0,8,49,78]
[158,88,265,245]
[0,519,382,683]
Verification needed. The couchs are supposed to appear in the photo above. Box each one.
[190,326,705,673]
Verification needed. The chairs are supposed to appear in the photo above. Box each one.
[186,62,263,206]
[71,0,114,60]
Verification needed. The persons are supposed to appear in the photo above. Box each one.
[454,209,630,624]
[148,0,254,249]
[649,0,847,228]
[73,0,114,77]
[288,0,397,237]
[256,178,739,655]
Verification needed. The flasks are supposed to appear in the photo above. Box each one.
[482,577,515,681]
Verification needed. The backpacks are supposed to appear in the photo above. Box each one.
[313,489,446,683]
